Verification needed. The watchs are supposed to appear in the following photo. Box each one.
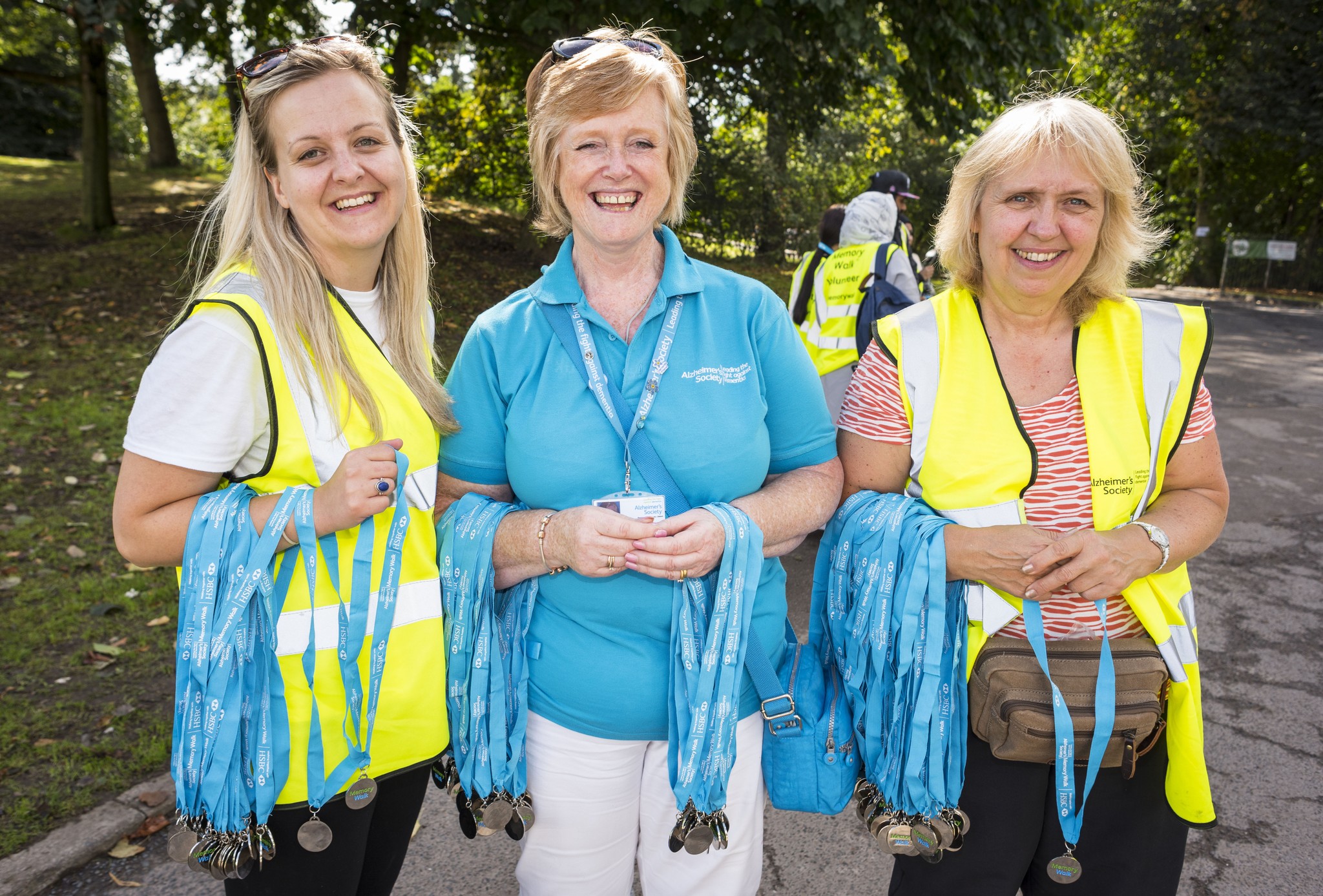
[1115,521,1170,574]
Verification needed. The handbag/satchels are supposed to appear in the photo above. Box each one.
[855,243,915,358]
[971,636,1168,782]
[758,641,862,815]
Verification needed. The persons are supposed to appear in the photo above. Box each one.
[112,32,452,896]
[440,22,846,896]
[782,167,937,533]
[831,90,1230,896]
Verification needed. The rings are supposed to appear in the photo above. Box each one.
[677,568,687,584]
[606,555,615,570]
[376,477,389,497]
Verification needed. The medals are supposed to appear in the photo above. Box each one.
[296,817,333,853]
[166,826,275,882]
[345,777,378,810]
[853,776,971,864]
[430,755,536,841]
[667,799,731,855]
[1047,856,1082,884]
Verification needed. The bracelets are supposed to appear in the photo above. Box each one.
[280,529,303,546]
[537,512,569,574]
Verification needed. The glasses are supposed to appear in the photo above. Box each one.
[552,35,663,65]
[237,35,349,113]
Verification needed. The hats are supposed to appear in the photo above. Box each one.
[873,169,922,201]
